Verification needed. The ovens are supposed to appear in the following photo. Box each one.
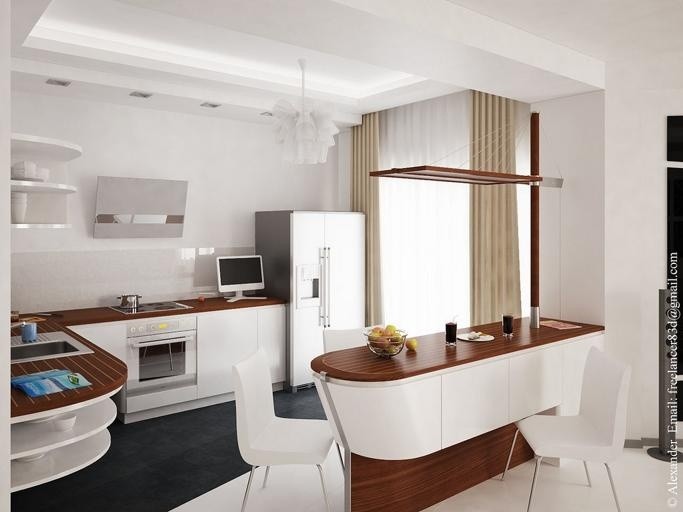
[126,329,198,398]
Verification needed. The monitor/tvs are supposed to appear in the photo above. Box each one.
[216,254,268,303]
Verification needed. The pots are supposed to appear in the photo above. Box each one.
[117,295,141,310]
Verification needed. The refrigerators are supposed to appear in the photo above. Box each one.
[254,210,367,394]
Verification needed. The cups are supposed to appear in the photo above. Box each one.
[501,312,515,338]
[21,322,37,344]
[11,192,28,224]
[445,321,457,347]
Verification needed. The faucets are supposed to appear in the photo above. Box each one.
[11,321,25,329]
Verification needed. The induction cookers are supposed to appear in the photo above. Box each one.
[108,301,194,316]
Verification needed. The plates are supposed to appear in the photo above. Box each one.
[457,334,494,342]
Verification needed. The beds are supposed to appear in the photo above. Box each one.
[311,315,605,511]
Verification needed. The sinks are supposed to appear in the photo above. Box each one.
[11,340,79,364]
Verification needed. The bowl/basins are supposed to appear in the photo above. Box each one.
[53,414,76,431]
[365,330,408,359]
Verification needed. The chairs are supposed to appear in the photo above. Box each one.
[322,328,369,355]
[500,346,632,512]
[232,346,344,511]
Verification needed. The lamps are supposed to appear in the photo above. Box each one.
[274,57,340,164]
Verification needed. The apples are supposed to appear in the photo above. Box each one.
[405,338,418,351]
[369,326,402,353]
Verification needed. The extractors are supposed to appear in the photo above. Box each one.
[94,176,188,241]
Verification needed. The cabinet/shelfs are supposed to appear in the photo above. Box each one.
[196,303,287,408]
[10,313,128,494]
[10,133,82,230]
